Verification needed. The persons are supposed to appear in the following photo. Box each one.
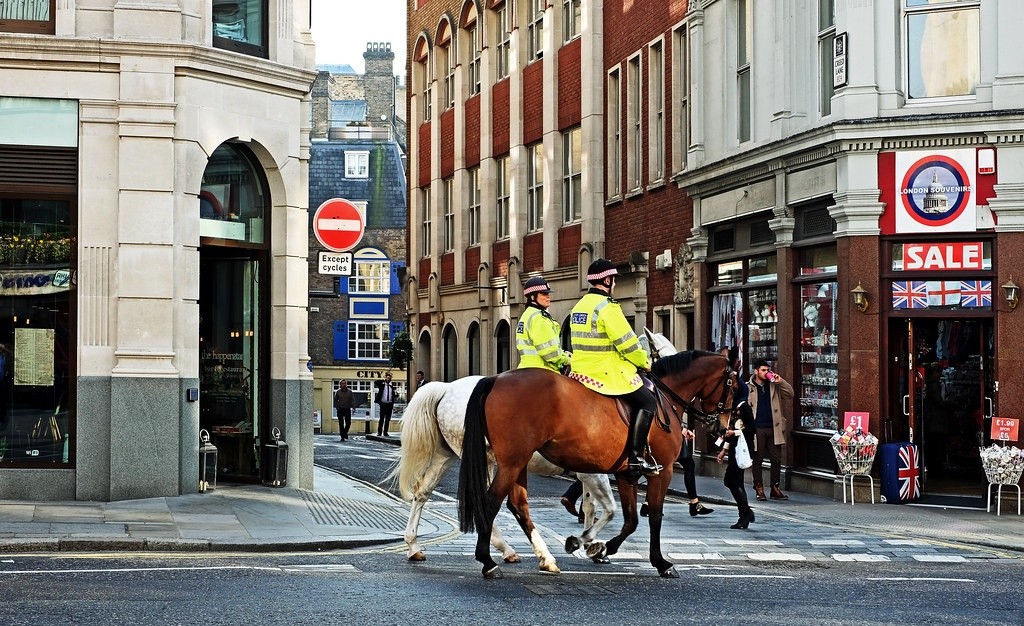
[333,379,356,441]
[745,360,794,501]
[374,374,395,436]
[560,481,599,525]
[717,411,755,529]
[640,425,714,517]
[568,258,663,472]
[515,278,572,375]
[416,371,428,391]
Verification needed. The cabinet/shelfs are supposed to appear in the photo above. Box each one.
[749,301,777,364]
[800,297,837,434]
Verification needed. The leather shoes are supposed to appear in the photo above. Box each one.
[578,517,599,523]
[561,497,579,516]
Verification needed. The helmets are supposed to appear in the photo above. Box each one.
[524,277,555,297]
[587,258,621,280]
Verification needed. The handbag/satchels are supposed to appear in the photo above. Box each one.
[735,430,753,469]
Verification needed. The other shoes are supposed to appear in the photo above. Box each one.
[377,434,381,436]
[640,503,665,516]
[689,502,714,516]
[731,511,755,529]
[384,434,389,436]
[342,429,348,439]
[341,438,344,441]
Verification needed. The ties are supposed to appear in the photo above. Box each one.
[386,383,389,401]
[418,382,421,386]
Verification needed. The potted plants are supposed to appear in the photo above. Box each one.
[389,333,414,373]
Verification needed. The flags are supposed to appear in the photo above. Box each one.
[926,281,961,306]
[892,281,928,309]
[961,280,992,307]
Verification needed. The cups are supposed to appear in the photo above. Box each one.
[764,372,775,382]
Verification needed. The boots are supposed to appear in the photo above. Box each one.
[770,480,789,500]
[753,480,767,501]
[627,409,664,474]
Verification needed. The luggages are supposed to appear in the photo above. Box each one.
[882,442,921,504]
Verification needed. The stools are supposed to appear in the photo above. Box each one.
[986,482,1022,516]
[843,474,875,506]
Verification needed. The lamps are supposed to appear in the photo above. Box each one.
[1001,275,1019,310]
[850,281,870,313]
[629,254,648,267]
[438,313,445,325]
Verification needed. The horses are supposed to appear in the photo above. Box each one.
[457,345,757,585]
[377,327,677,560]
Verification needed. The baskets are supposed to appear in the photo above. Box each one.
[979,443,1024,484]
[832,444,876,474]
